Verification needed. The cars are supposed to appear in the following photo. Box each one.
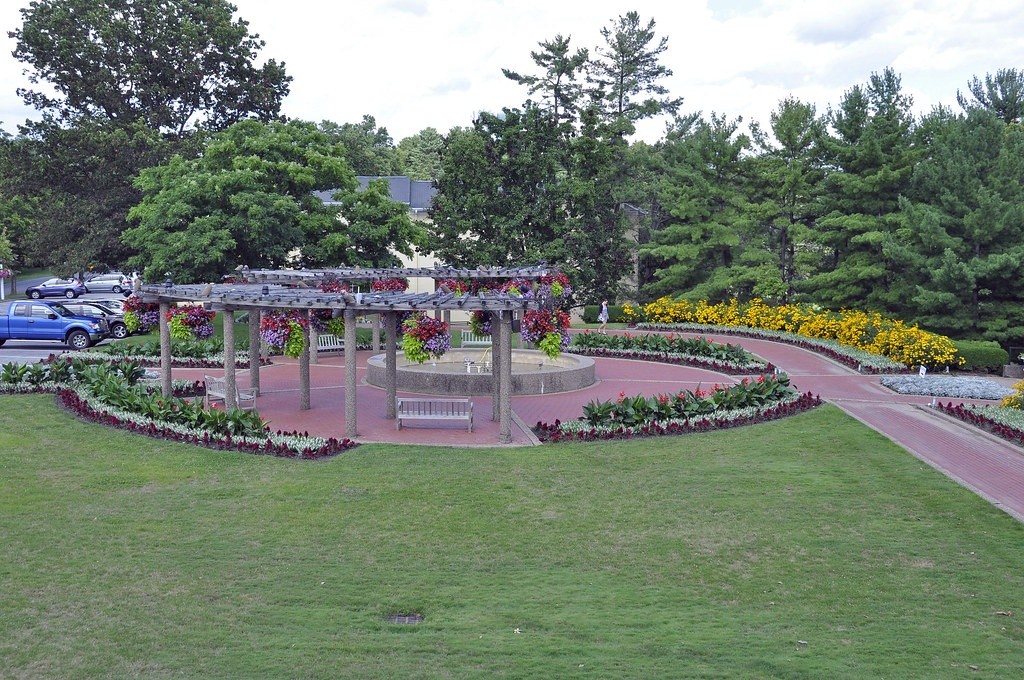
[108,298,132,315]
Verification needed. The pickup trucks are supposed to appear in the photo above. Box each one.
[1,299,111,349]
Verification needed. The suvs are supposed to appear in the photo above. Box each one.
[83,273,134,294]
[25,276,86,299]
[57,301,133,338]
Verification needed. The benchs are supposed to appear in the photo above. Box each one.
[204,374,259,412]
[396,398,474,433]
[308,334,345,357]
[461,329,492,348]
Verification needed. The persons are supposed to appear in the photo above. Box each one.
[597,298,610,335]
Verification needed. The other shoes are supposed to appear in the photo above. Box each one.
[602,332,607,335]
[597,329,601,334]
